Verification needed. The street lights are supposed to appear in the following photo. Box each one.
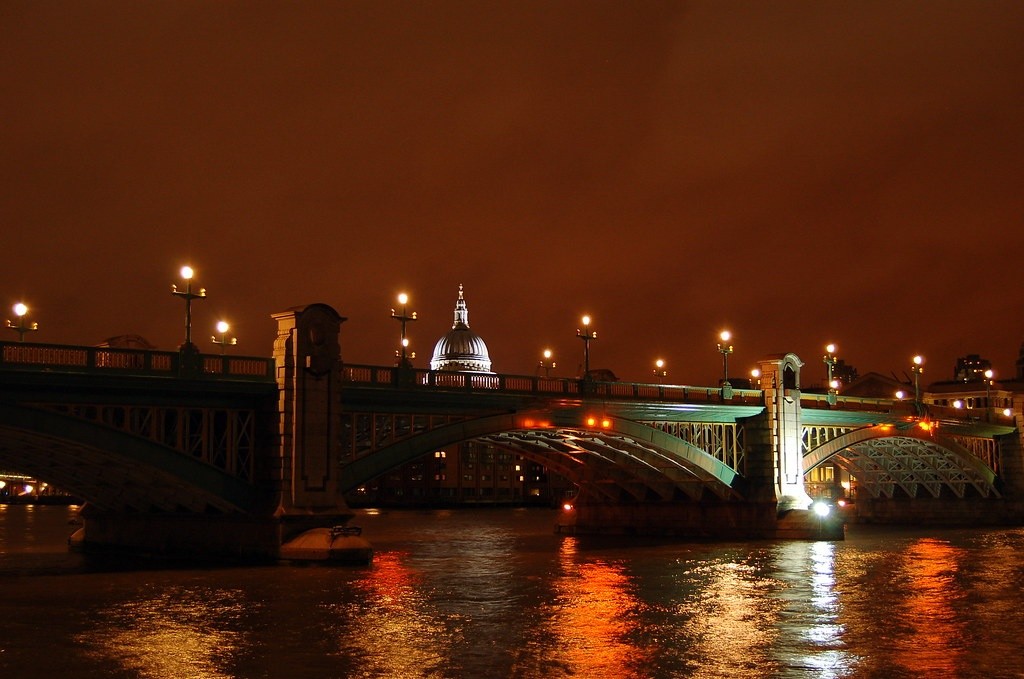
[6,304,39,363]
[748,369,760,389]
[171,266,208,352]
[650,359,669,383]
[822,344,837,388]
[390,293,418,366]
[210,321,238,353]
[395,339,416,366]
[576,316,597,373]
[985,371,993,408]
[716,331,734,386]
[541,349,557,377]
[911,355,923,401]
